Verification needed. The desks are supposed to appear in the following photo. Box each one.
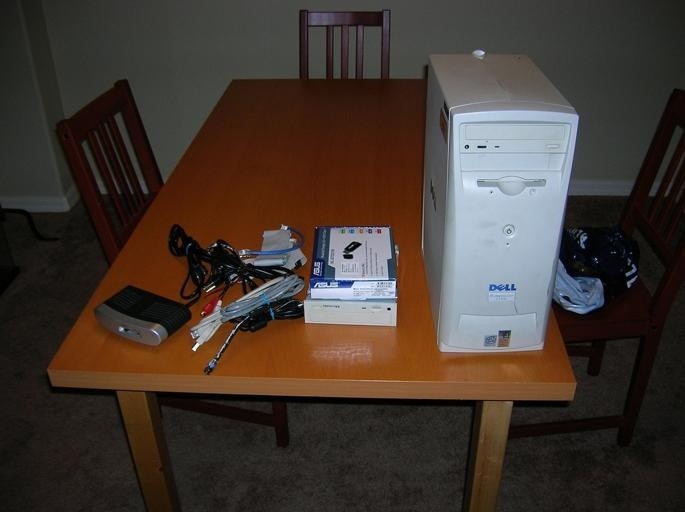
[48,75,577,512]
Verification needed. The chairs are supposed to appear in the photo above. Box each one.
[298,8,391,82]
[56,78,290,449]
[509,89,685,446]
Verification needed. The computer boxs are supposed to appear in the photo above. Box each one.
[421,52,581,353]
